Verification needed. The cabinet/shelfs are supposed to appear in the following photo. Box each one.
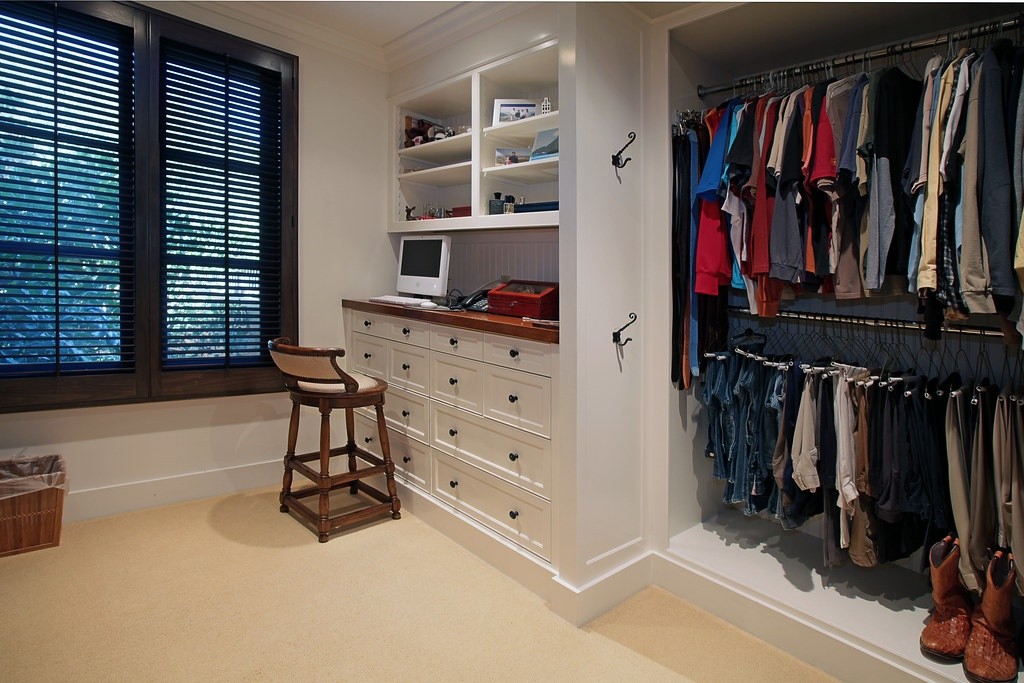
[382,40,566,226]
[341,293,560,572]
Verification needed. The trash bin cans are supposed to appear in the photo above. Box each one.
[0,453,68,558]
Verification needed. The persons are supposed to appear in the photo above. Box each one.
[509,107,532,121]
[502,150,519,165]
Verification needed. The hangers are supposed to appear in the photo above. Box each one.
[674,10,1022,136]
[706,306,1022,409]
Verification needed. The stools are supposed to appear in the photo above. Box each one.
[266,336,404,542]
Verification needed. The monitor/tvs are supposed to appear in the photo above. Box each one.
[397,235,452,296]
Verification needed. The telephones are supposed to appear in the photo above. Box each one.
[461,288,493,312]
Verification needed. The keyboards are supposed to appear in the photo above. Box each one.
[369,295,431,307]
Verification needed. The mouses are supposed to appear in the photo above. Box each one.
[421,302,438,309]
